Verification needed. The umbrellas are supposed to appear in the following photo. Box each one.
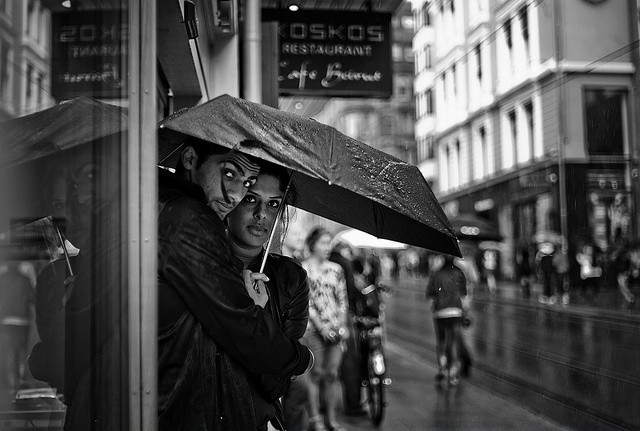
[1,95,128,178]
[335,228,408,251]
[447,214,502,243]
[164,94,463,294]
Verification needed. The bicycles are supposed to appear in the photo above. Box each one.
[347,281,397,428]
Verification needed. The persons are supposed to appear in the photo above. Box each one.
[300,228,349,431]
[63,145,124,430]
[425,253,467,381]
[35,160,92,430]
[327,243,370,420]
[155,137,314,429]
[222,168,308,430]
